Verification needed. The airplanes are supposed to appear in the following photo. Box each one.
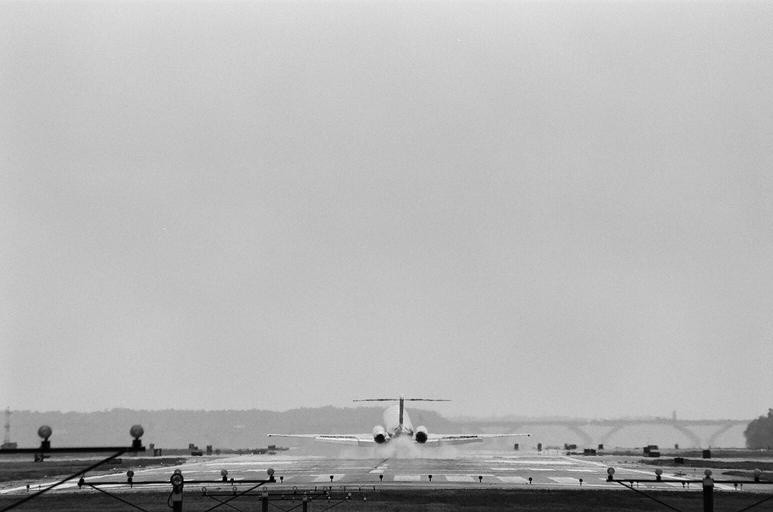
[261,393,542,447]
